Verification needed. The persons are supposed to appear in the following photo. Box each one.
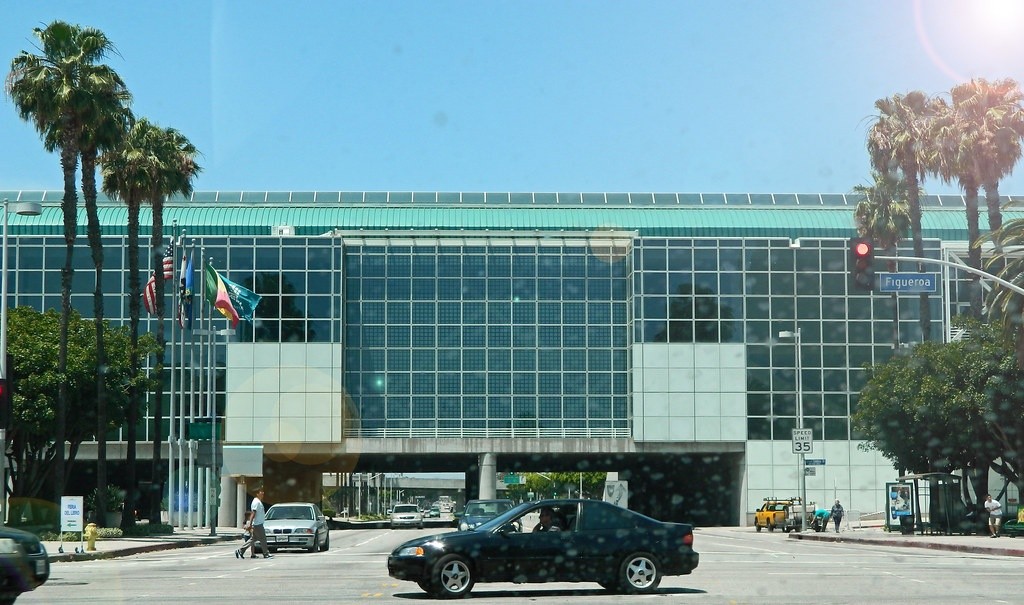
[810,509,831,532]
[892,487,910,511]
[513,506,561,532]
[234,488,274,559]
[241,512,259,559]
[985,494,1003,538]
[829,500,845,533]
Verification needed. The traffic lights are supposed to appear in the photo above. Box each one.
[849,234,875,295]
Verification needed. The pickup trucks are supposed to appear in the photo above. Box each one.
[754,498,808,532]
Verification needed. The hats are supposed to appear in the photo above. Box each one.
[251,487,264,494]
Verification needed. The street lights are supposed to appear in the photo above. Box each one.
[778,325,806,533]
[0,197,43,523]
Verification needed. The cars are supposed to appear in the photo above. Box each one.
[421,508,441,518]
[391,503,422,529]
[1,523,51,605]
[387,497,700,600]
[249,502,331,554]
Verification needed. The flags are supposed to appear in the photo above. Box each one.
[142,234,263,329]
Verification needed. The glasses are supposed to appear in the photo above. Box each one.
[539,514,548,518]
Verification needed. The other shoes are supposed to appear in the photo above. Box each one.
[238,549,244,559]
[235,549,239,558]
[251,554,260,558]
[264,553,274,558]
